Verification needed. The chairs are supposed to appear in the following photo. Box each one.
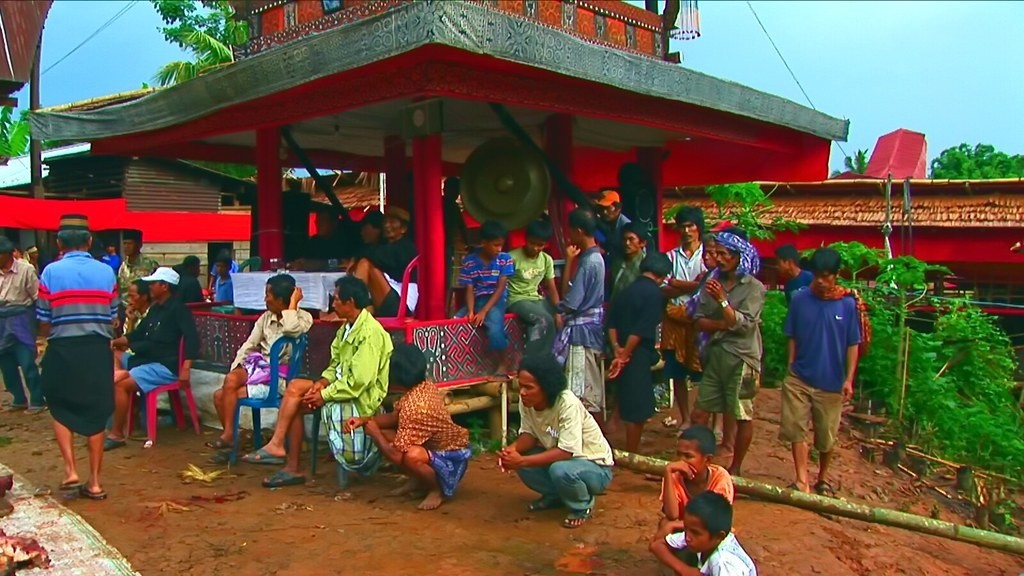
[232,334,305,458]
[125,333,200,445]
[211,256,262,314]
[308,405,375,491]
[373,255,420,322]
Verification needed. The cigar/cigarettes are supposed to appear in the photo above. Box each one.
[350,425,354,440]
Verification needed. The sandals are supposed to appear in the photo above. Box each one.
[563,507,591,528]
[526,497,561,511]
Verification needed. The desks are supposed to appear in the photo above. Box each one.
[233,272,346,317]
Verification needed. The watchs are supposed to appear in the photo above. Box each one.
[718,300,729,309]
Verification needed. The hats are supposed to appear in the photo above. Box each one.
[123,228,143,240]
[593,190,620,206]
[141,267,180,285]
[388,206,410,222]
[59,214,88,230]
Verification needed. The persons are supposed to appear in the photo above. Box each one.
[648,491,758,576]
[247,169,487,325]
[35,214,121,499]
[653,425,732,541]
[778,247,861,500]
[203,275,313,468]
[341,339,471,509]
[240,274,394,487]
[496,353,614,529]
[0,219,245,451]
[774,244,816,312]
[447,187,767,477]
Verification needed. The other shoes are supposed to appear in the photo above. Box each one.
[24,407,47,415]
[9,403,28,411]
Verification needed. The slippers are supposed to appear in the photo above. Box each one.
[208,450,232,465]
[205,436,233,449]
[786,483,799,491]
[262,469,304,487]
[59,480,82,490]
[815,480,835,499]
[80,482,106,499]
[239,447,284,465]
[670,427,685,438]
[104,437,126,451]
